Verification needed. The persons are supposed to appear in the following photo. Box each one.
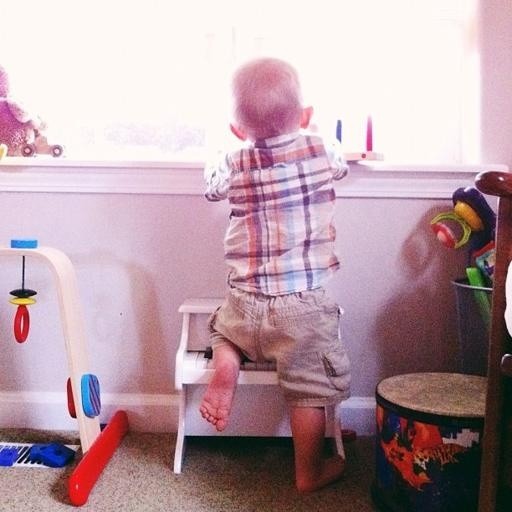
[198,57,348,493]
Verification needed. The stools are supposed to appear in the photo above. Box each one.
[371,371,487,512]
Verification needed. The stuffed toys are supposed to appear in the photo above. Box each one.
[0,70,62,157]
